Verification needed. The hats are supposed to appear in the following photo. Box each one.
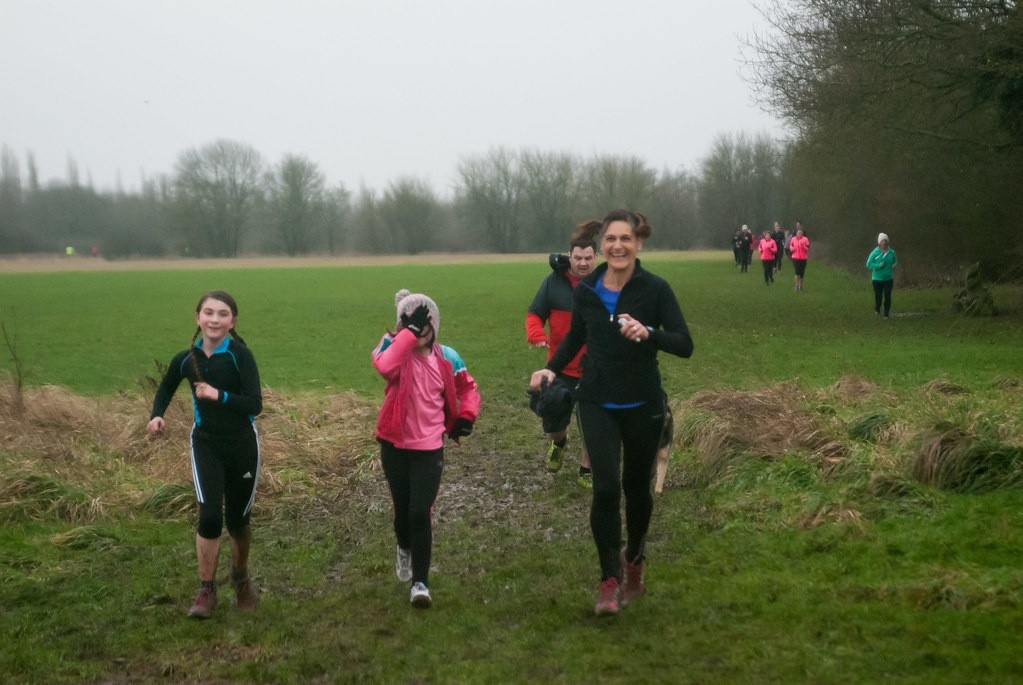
[878,233,889,244]
[395,289,439,342]
[742,225,748,230]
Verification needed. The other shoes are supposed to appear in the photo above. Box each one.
[884,316,888,320]
[875,311,879,315]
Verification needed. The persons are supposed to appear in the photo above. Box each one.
[758,232,778,284]
[525,235,612,490]
[370,289,479,605]
[790,231,808,294]
[764,220,809,271]
[867,233,899,319]
[145,291,263,619]
[731,223,756,273]
[530,209,695,616]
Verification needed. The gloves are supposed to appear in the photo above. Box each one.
[400,304,432,338]
[449,418,472,439]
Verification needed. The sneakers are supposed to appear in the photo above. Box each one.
[232,573,260,609]
[396,545,413,582]
[577,473,593,489]
[545,433,567,472]
[621,545,644,598]
[595,576,623,615]
[409,582,432,602]
[187,587,216,618]
